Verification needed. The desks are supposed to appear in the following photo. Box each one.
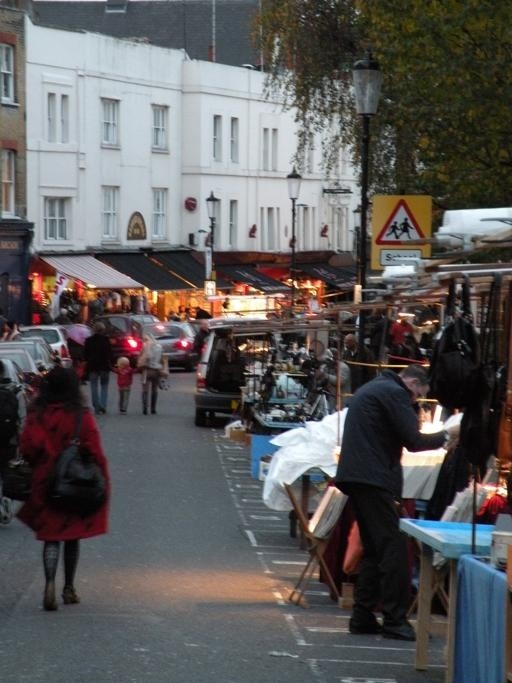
[250,402,306,428]
[320,447,448,499]
[398,515,498,683]
[456,553,511,681]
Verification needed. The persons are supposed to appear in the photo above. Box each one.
[168,305,214,325]
[19,366,110,610]
[0,360,30,524]
[0,321,23,344]
[287,333,378,413]
[112,357,138,413]
[137,333,166,414]
[334,363,463,641]
[84,321,114,415]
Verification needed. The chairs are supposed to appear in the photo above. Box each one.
[406,479,486,617]
[283,479,349,610]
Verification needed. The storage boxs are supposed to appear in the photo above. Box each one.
[259,456,271,481]
[229,426,245,441]
[243,432,252,445]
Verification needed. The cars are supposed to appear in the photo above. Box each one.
[0,311,194,405]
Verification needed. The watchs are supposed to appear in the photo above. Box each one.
[443,430,450,441]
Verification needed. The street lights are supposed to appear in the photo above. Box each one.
[350,50,383,349]
[203,190,222,299]
[284,166,305,328]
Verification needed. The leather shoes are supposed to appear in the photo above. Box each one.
[350,620,382,635]
[381,626,416,641]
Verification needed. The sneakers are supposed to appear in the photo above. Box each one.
[44,588,58,610]
[61,591,81,605]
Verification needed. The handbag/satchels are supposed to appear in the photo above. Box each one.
[44,440,105,519]
[431,312,479,409]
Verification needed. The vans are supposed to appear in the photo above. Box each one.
[194,319,283,429]
